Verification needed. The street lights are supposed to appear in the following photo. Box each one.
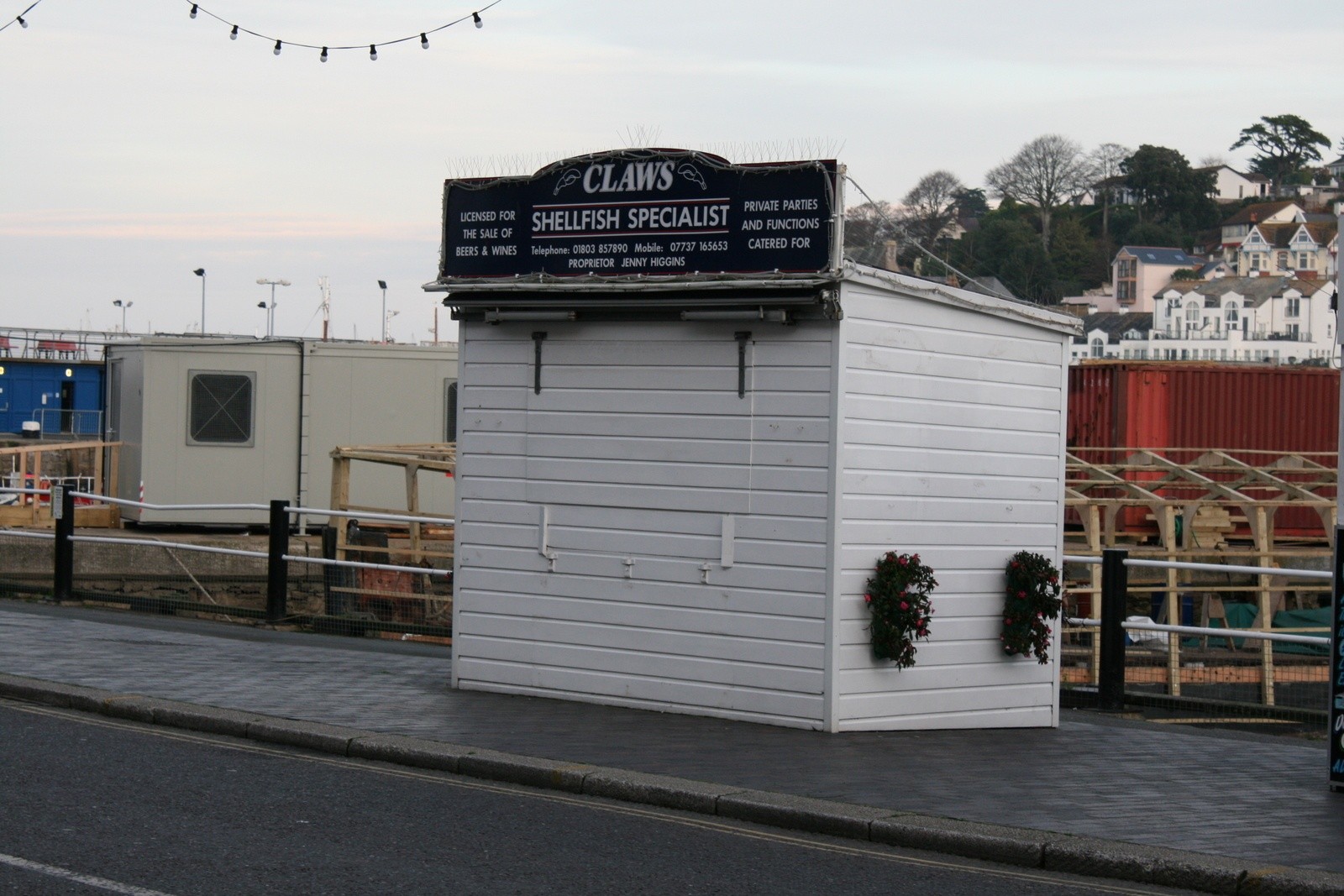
[259,276,292,337]
[257,301,276,337]
[384,310,402,345]
[113,298,135,333]
[378,280,390,342]
[194,268,209,334]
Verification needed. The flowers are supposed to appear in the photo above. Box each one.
[999,549,1062,665]
[864,548,939,672]
